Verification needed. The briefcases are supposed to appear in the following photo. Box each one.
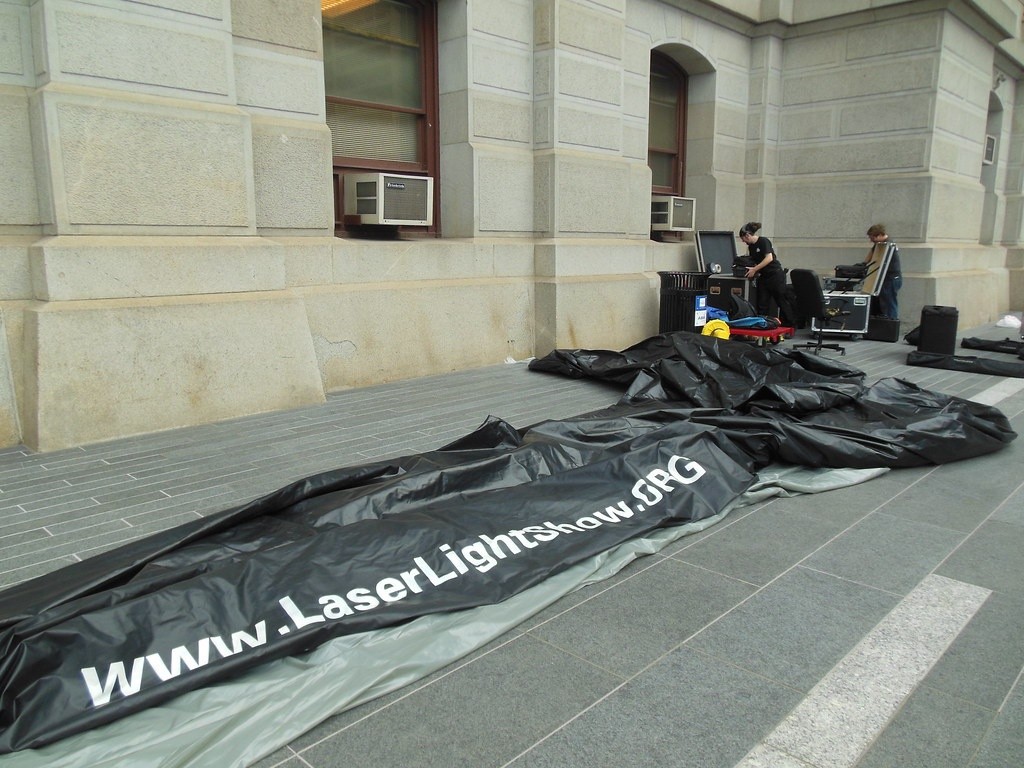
[863,314,899,343]
[918,304,958,355]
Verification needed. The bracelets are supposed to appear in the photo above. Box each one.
[755,266,758,271]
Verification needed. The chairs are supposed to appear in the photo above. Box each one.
[791,268,851,357]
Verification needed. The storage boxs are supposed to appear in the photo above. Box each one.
[694,230,758,308]
[811,242,897,334]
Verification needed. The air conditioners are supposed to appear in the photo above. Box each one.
[651,196,696,232]
[984,134,996,166]
[344,172,433,227]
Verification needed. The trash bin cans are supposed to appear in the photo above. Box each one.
[657,271,712,335]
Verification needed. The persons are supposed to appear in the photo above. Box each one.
[863,223,903,320]
[732,221,786,341]
